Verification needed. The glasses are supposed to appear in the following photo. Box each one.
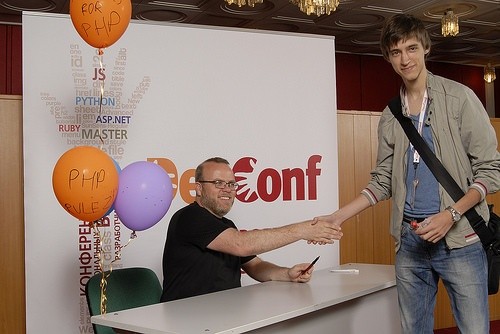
[196,178,240,191]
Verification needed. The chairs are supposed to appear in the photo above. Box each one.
[85,267,163,334]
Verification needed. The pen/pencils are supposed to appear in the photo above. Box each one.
[330,269,360,273]
[302,255,320,276]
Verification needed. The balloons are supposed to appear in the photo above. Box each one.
[93,157,122,222]
[115,160,173,239]
[52,146,118,228]
[70,0,132,55]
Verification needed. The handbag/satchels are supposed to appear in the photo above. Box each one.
[480,201,500,296]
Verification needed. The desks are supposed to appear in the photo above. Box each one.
[90,263,403,334]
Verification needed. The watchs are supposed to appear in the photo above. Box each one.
[445,206,461,224]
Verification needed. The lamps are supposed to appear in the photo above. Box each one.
[441,11,459,37]
[227,0,262,8]
[484,64,496,82]
[291,0,340,17]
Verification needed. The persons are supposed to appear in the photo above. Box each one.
[308,13,499,334]
[160,157,343,303]
